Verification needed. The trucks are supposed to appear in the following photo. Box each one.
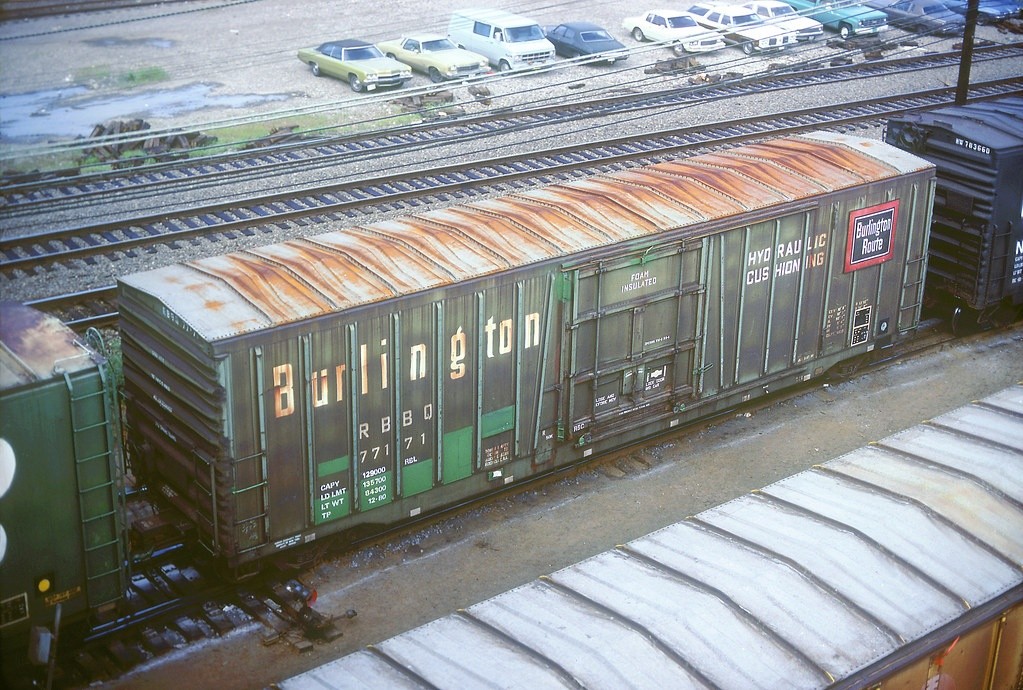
[776,0,890,41]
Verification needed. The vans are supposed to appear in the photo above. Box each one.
[446,8,556,79]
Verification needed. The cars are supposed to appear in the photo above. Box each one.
[546,21,630,67]
[938,0,1021,27]
[298,40,413,92]
[742,1,823,44]
[377,34,491,85]
[622,8,726,56]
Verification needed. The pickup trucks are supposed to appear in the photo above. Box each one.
[683,2,797,56]
[861,0,967,36]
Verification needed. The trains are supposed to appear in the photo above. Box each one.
[0,91,1023,643]
